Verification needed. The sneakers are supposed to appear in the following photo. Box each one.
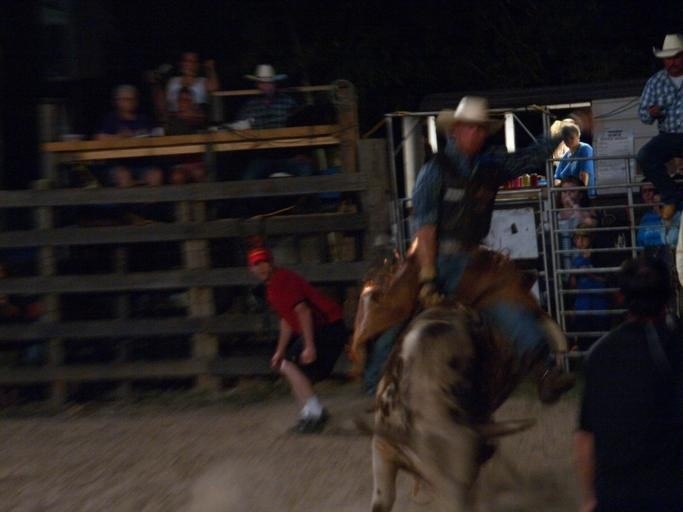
[288,407,331,435]
[538,365,576,405]
[659,201,676,221]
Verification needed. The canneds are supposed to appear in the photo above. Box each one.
[504,173,538,190]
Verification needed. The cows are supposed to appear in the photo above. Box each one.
[347,303,540,512]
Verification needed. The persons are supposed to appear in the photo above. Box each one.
[92,49,312,188]
[247,248,346,436]
[362,33,682,509]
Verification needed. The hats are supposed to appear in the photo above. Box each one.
[433,94,507,140]
[649,32,682,61]
[239,62,290,84]
[244,244,273,267]
